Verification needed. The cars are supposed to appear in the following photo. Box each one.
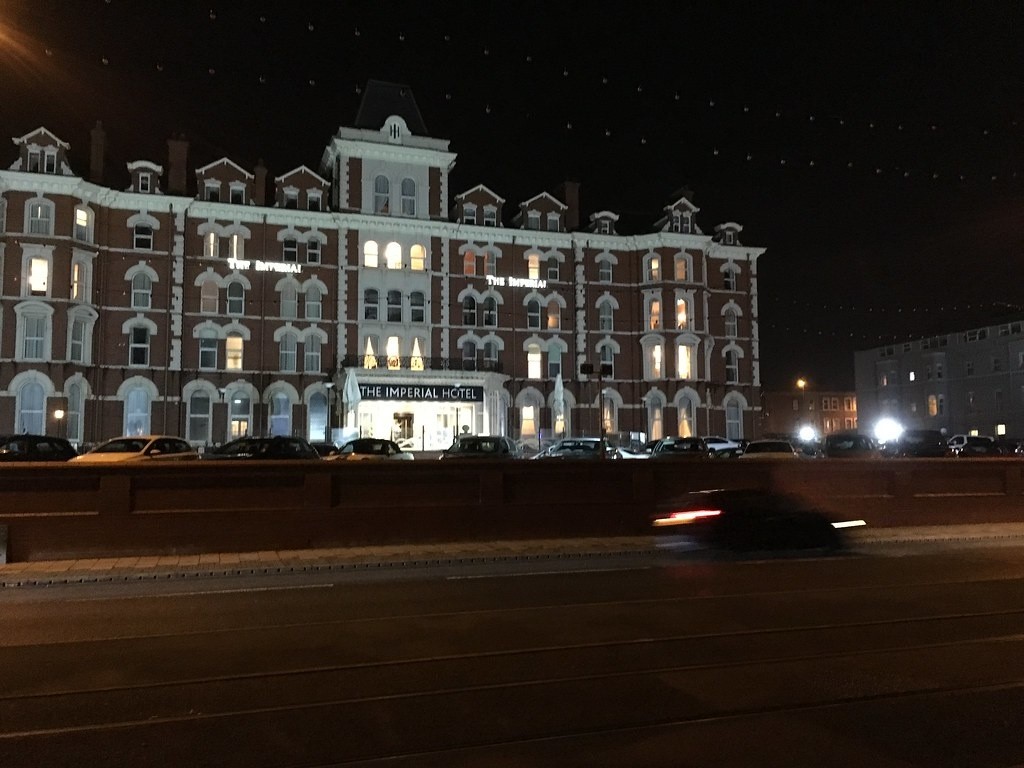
[649,489,844,556]
[945,432,1003,457]
[199,433,320,466]
[899,428,949,461]
[548,436,613,463]
[530,445,557,461]
[339,437,403,460]
[819,434,882,464]
[65,434,200,465]
[604,435,831,461]
[992,437,1024,457]
[1,434,79,465]
[438,435,524,465]
[308,443,340,459]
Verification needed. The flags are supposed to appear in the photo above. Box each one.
[555,373,563,402]
[342,367,362,413]
